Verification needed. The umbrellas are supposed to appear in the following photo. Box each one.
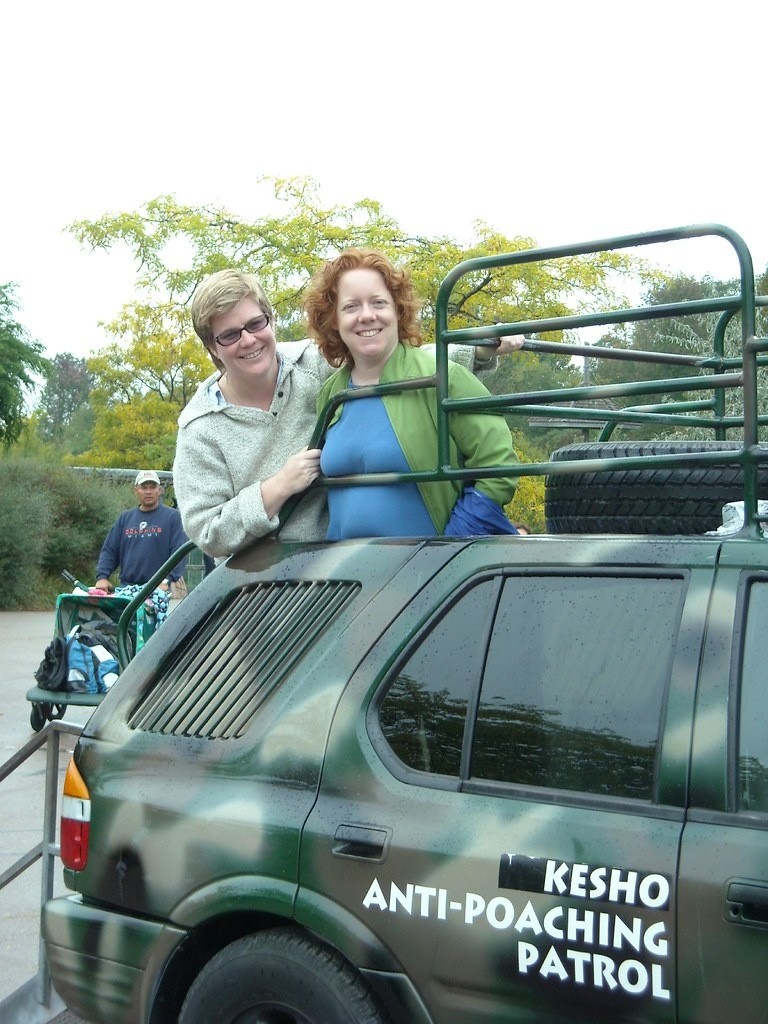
[62,569,90,592]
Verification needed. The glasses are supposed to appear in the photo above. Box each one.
[212,312,270,347]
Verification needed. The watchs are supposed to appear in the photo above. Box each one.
[162,578,171,586]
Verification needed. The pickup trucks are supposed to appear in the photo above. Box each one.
[40,220,768,1024]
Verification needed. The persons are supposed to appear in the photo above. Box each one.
[173,270,525,565]
[304,252,520,537]
[515,524,530,536]
[95,469,189,599]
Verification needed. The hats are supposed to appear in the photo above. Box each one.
[134,470,161,485]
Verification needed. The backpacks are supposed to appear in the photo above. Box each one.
[34,620,133,694]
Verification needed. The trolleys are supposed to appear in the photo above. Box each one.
[26,568,171,733]
